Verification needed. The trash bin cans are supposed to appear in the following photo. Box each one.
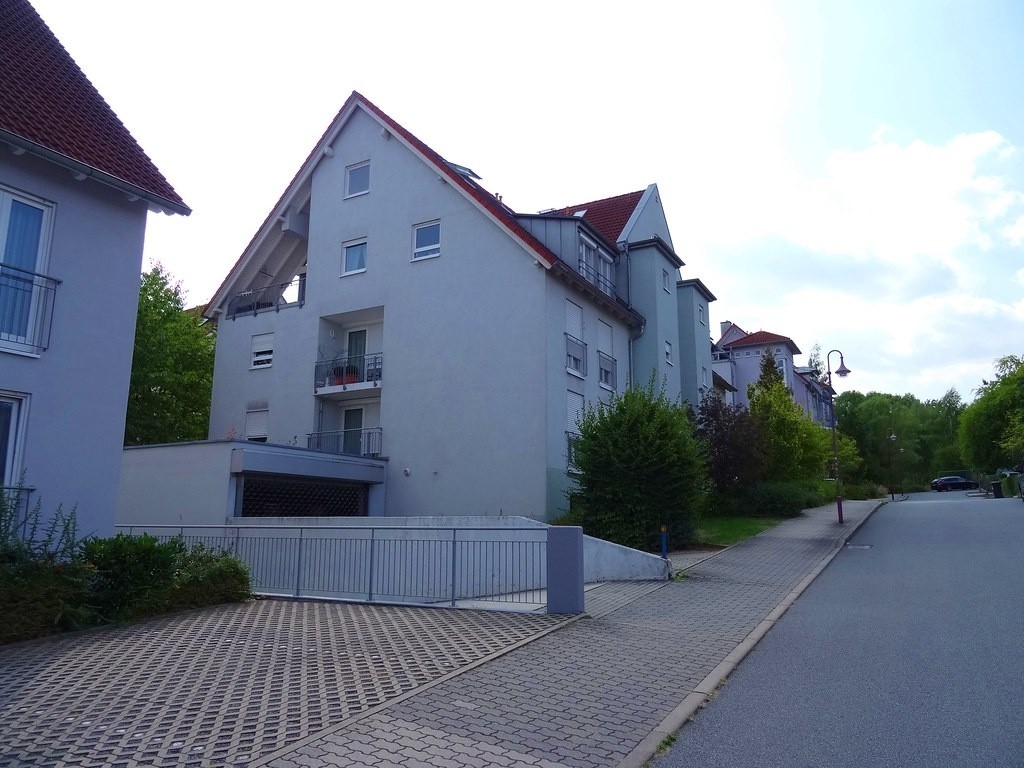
[991,481,1004,498]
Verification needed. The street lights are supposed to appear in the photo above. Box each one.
[896,448,905,497]
[886,427,897,500]
[828,348,852,527]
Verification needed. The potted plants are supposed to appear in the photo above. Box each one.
[346,365,358,383]
[333,366,342,385]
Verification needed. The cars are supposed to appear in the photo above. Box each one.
[930,476,980,492]
